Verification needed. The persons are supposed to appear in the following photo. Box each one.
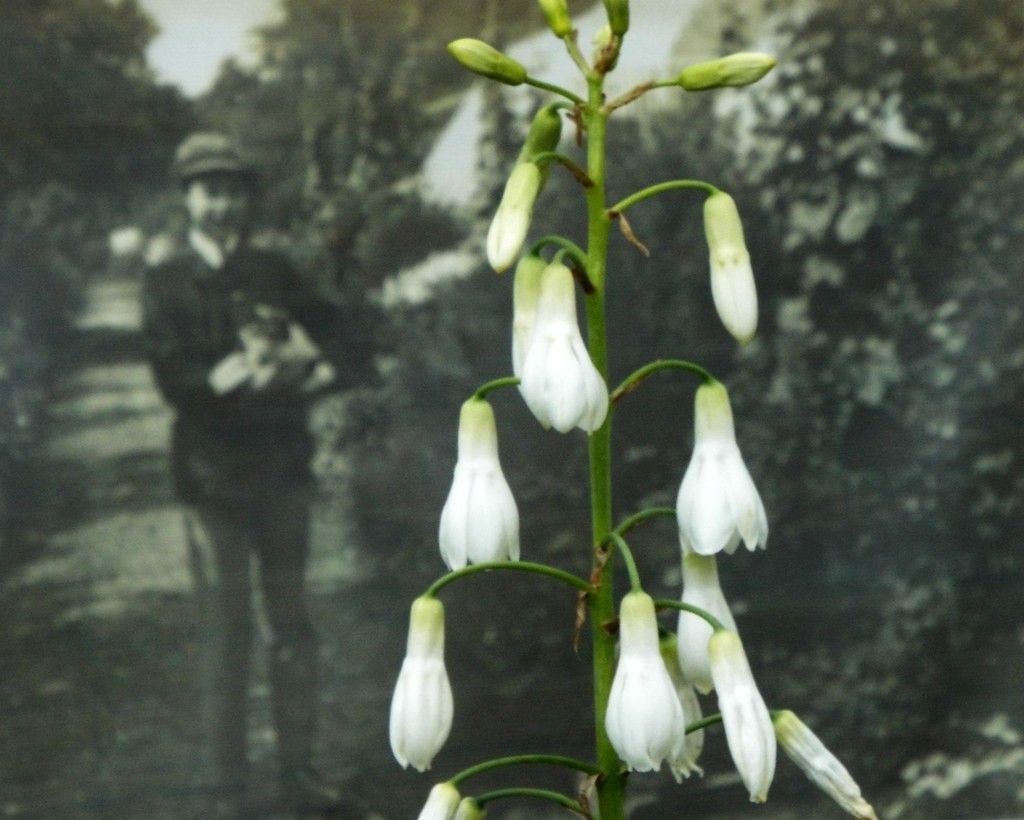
[143,129,385,810]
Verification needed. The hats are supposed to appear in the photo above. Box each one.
[177,132,243,178]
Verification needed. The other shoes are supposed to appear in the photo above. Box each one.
[278,760,352,813]
[211,776,246,819]
